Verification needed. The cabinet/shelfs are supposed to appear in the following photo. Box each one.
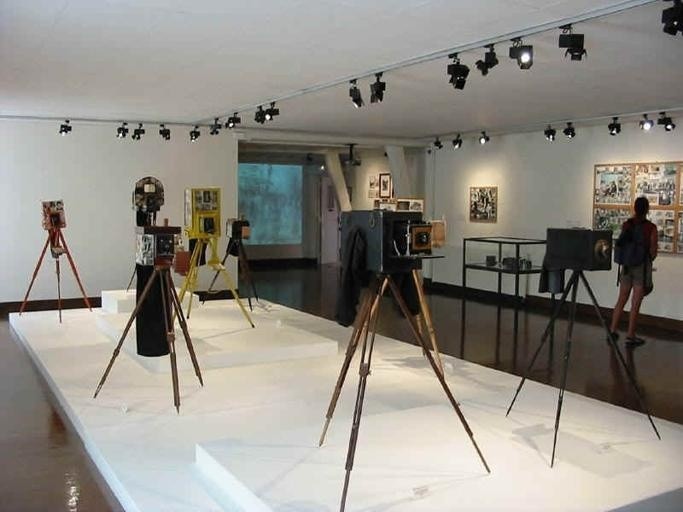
[464,236,555,317]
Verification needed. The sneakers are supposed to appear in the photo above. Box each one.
[607,331,619,345]
[625,336,644,349]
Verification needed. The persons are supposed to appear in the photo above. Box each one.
[607,197,658,345]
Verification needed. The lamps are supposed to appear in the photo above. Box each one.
[58,0,683,144]
[416,107,683,149]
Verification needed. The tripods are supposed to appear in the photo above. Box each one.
[18,231,92,322]
[96,228,258,412]
[316,269,491,512]
[355,270,462,406]
[507,272,660,468]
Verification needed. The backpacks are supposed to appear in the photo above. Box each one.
[620,218,650,268]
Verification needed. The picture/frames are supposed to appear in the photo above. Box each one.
[469,186,497,223]
[379,173,391,198]
[592,161,683,257]
